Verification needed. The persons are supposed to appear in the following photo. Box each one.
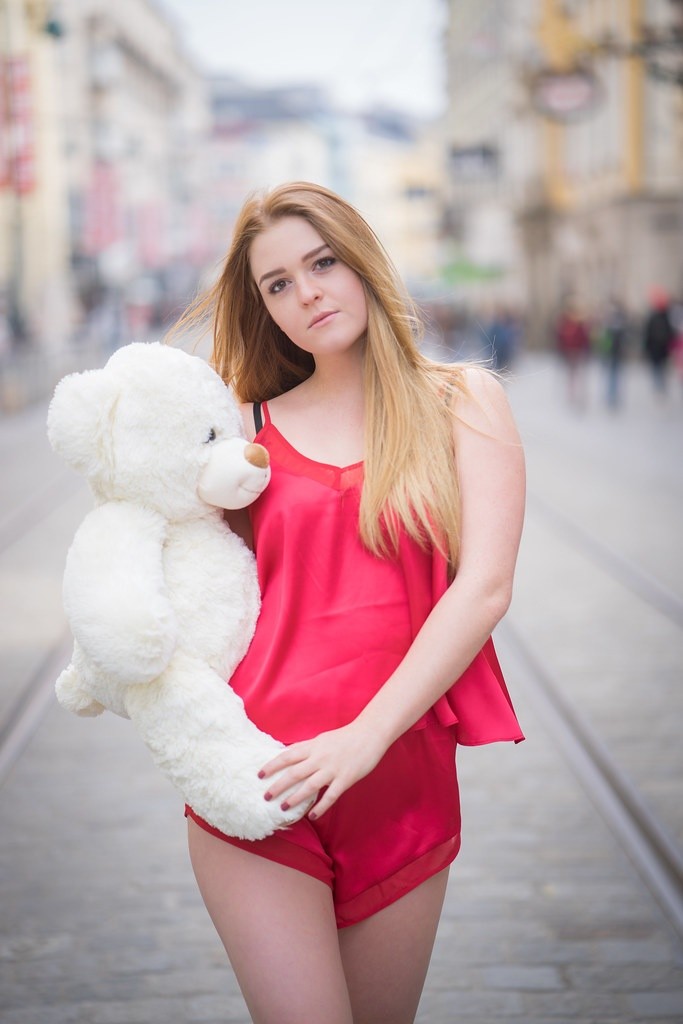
[441,304,513,369]
[184,181,527,1024]
[561,301,673,404]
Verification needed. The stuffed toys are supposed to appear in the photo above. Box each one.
[47,341,318,841]
